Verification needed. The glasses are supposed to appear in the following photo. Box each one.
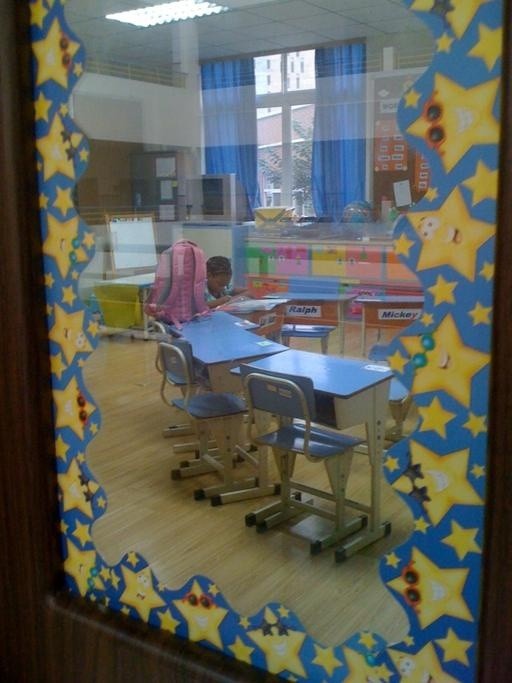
[215,281,232,289]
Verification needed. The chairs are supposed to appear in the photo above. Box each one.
[154,322,202,438]
[281,279,339,354]
[159,339,259,501]
[238,363,369,555]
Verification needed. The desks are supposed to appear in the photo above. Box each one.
[229,350,394,563]
[168,312,291,393]
[355,292,424,358]
[243,238,417,322]
[217,291,359,354]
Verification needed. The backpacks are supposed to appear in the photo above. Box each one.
[144,239,211,331]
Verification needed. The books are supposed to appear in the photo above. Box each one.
[215,293,242,310]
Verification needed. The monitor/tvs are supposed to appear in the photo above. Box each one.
[182,173,247,226]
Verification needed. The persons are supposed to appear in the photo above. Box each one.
[203,254,250,310]
